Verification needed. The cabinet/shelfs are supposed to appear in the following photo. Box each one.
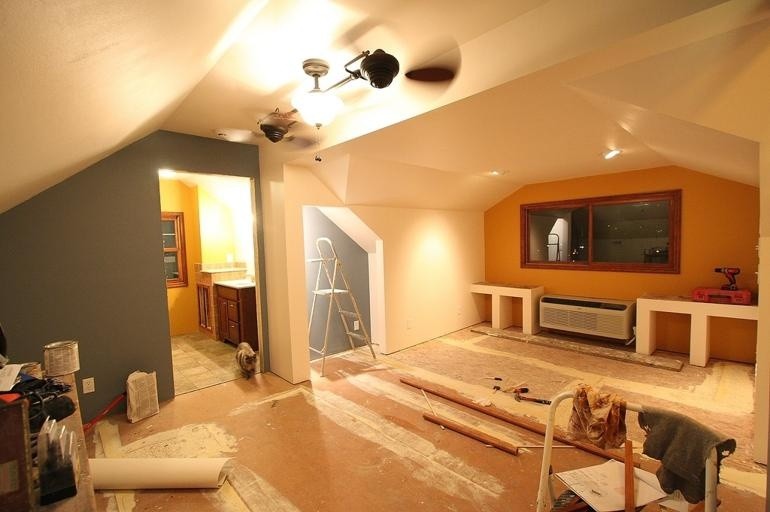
[198,283,220,341]
[217,285,258,351]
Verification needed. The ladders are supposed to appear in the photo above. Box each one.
[308,237,376,378]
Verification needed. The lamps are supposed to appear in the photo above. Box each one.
[261,49,407,162]
[602,150,621,159]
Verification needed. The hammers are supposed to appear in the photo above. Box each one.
[513,392,552,405]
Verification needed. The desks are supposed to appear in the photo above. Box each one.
[31,370,97,511]
[636,296,758,366]
[471,281,544,335]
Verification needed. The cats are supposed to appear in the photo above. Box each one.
[236,342,257,379]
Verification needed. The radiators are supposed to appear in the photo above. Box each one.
[540,295,635,340]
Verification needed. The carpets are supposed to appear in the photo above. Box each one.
[171,332,261,395]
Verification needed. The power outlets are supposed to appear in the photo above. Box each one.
[81,377,95,394]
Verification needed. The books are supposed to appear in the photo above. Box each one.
[553,458,667,512]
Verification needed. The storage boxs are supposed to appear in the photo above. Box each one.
[0,397,32,512]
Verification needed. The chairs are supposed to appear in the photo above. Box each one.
[535,392,721,512]
